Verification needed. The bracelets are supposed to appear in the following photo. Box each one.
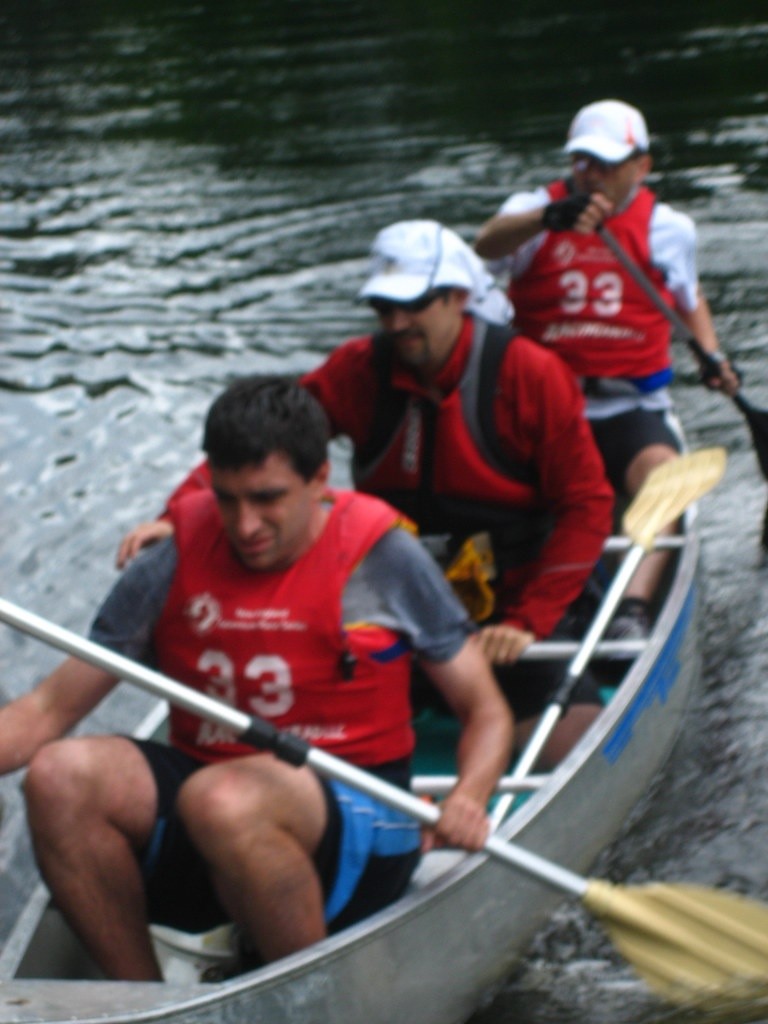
[701,351,728,366]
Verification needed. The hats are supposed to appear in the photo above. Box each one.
[354,219,473,301]
[564,100,650,162]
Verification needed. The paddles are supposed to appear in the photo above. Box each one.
[0,593,768,1006]
[591,227,768,475]
[478,447,732,838]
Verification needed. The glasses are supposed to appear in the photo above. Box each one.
[368,289,445,314]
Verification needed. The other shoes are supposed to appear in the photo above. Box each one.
[602,617,648,642]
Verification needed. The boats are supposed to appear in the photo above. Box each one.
[4,403,705,1023]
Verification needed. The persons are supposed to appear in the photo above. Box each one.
[114,220,617,782]
[0,379,515,978]
[472,100,742,680]
